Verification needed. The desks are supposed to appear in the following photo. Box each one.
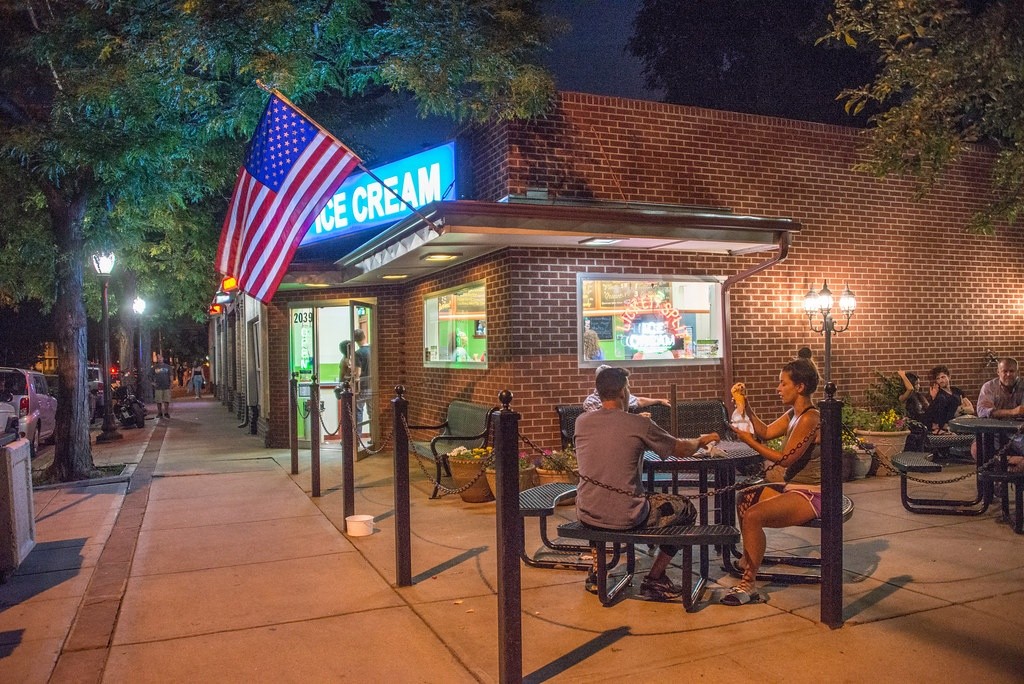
[948,416,1024,515]
[640,442,766,582]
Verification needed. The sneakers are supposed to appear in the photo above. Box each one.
[640,570,683,600]
[584,566,609,594]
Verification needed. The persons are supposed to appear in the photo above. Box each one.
[450,331,473,361]
[572,368,719,600]
[583,330,605,360]
[583,364,671,417]
[897,366,975,435]
[147,353,173,418]
[339,330,373,446]
[970,358,1024,499]
[178,360,210,400]
[720,346,825,606]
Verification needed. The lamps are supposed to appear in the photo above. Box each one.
[209,277,236,316]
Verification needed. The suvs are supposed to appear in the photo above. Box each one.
[0,389,18,447]
[0,367,59,457]
[88,367,105,409]
[45,374,99,425]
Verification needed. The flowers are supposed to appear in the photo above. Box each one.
[447,446,580,476]
[768,408,917,454]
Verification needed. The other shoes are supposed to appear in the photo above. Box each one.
[992,481,1003,502]
[368,439,373,444]
[156,412,162,418]
[164,412,170,418]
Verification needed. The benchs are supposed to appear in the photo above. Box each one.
[407,400,500,499]
[518,400,854,612]
[891,433,1024,533]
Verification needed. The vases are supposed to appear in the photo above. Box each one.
[841,446,854,480]
[485,466,535,498]
[535,467,579,505]
[852,447,876,479]
[448,458,492,502]
[854,429,910,477]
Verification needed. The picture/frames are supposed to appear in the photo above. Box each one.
[438,287,486,315]
[584,280,672,310]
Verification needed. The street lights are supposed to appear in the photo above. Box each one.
[92,251,123,444]
[132,295,147,398]
[804,279,856,398]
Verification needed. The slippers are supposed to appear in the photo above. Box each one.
[720,560,745,575]
[720,586,759,606]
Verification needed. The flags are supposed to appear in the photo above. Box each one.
[213,91,360,306]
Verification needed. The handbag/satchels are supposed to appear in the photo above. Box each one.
[187,381,194,394]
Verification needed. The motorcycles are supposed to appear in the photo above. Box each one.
[109,372,147,429]
